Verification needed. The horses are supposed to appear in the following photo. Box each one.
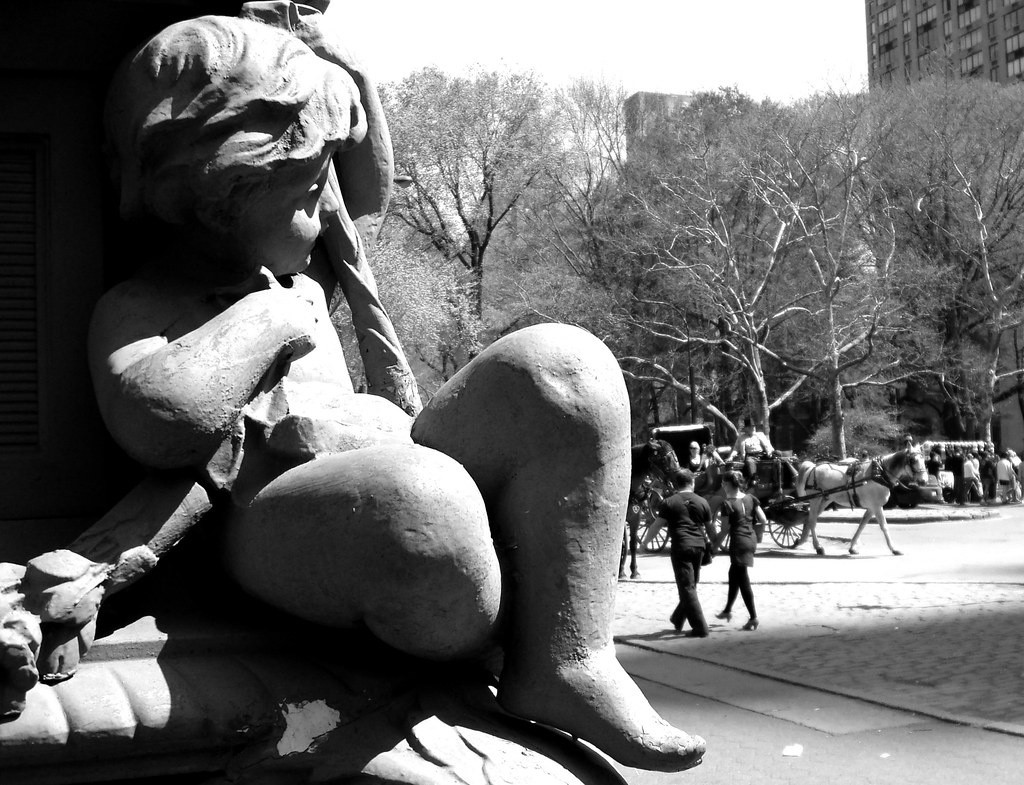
[618,437,681,580]
[795,440,926,555]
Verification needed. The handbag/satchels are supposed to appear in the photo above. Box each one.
[701,543,711,565]
[753,496,765,543]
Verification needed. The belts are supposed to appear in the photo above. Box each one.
[749,452,764,456]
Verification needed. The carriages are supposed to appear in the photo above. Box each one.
[631,421,930,555]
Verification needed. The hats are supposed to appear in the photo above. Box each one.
[740,417,757,430]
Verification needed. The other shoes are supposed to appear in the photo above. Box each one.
[670,617,683,631]
[752,473,761,484]
[769,478,777,483]
[685,629,706,637]
[980,498,989,506]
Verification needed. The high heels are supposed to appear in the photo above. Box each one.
[715,611,731,622]
[743,617,758,630]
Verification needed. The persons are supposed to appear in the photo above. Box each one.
[684,420,772,484]
[88,14,707,774]
[925,445,1024,506]
[640,470,717,638]
[710,471,765,630]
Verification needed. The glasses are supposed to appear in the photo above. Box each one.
[689,447,697,450]
[721,482,727,487]
